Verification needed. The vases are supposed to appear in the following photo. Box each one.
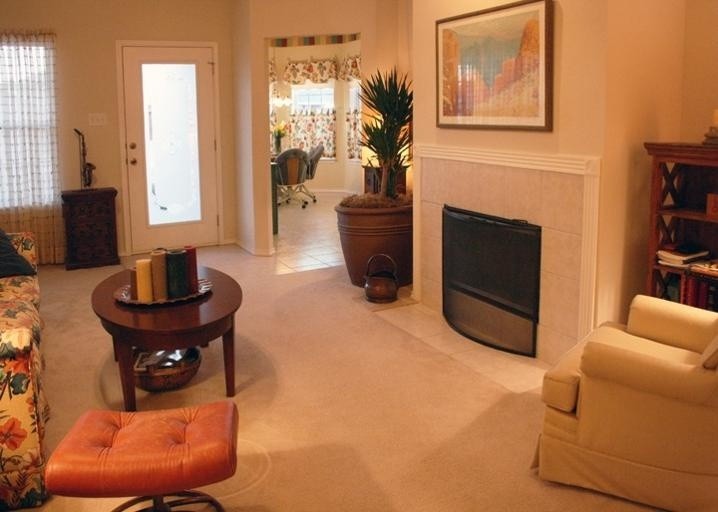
[275,134,283,156]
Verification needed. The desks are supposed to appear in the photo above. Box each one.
[91,263,243,411]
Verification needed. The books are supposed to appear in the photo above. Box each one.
[656,244,709,268]
[692,260,718,275]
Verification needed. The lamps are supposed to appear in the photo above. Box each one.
[268,48,293,110]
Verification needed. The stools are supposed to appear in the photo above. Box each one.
[42,400,241,512]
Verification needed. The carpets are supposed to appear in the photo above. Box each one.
[36,243,665,511]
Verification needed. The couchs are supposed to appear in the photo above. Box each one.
[0,231,50,512]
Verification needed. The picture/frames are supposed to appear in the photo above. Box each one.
[433,0,556,135]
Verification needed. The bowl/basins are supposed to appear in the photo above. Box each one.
[129,346,202,393]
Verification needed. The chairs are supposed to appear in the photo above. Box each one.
[288,140,325,205]
[529,290,718,512]
[275,149,311,211]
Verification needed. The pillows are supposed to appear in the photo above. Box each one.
[0,229,37,279]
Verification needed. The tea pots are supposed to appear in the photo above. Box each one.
[362,253,400,303]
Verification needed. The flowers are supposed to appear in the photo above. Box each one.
[269,119,295,149]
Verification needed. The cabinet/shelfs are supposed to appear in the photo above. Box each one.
[60,185,121,274]
[270,161,278,236]
[361,161,411,195]
[642,140,717,312]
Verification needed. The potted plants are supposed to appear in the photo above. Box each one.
[332,68,413,289]
